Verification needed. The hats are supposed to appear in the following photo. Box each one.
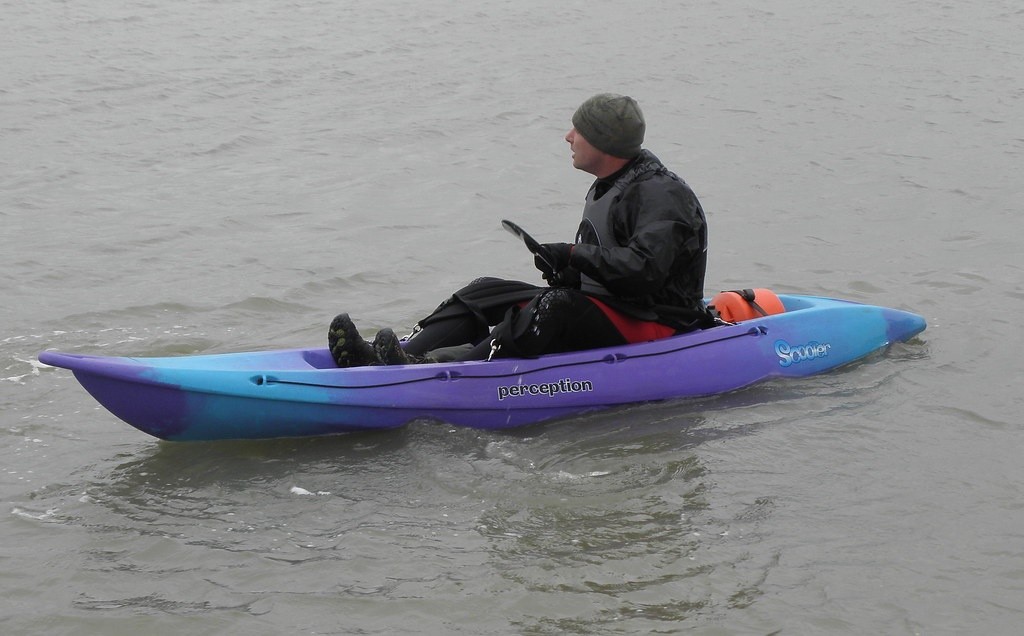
[572,93,645,158]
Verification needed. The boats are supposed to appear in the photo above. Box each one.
[35,288,929,443]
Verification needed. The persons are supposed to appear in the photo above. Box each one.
[327,92,709,368]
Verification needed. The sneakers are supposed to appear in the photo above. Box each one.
[372,328,426,366]
[327,313,373,367]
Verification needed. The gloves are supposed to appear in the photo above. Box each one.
[534,243,576,273]
